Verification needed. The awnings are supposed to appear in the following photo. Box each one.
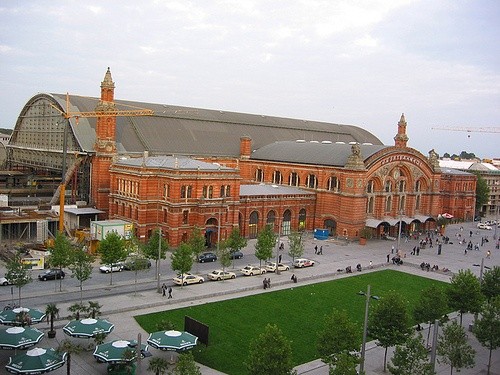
[416,216,436,223]
[365,219,393,228]
[382,216,406,226]
[401,217,422,225]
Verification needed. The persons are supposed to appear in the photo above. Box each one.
[387,220,500,272]
[346,260,374,273]
[168,286,172,299]
[279,255,281,263]
[263,278,270,289]
[315,245,322,255]
[281,243,284,249]
[291,274,296,283]
[162,283,167,296]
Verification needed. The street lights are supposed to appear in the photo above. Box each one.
[397,215,405,249]
[355,285,380,375]
[490,198,500,240]
[473,258,492,334]
[156,227,166,284]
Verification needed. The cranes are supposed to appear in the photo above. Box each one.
[58,92,157,234]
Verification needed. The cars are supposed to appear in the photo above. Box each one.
[173,272,205,286]
[241,266,266,276]
[124,259,151,271]
[266,262,290,272]
[99,262,125,274]
[207,270,236,281]
[294,258,315,268]
[196,252,217,263]
[223,250,243,260]
[0,274,29,286]
[38,268,65,281]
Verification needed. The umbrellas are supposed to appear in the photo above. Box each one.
[147,330,198,360]
[0,307,46,327]
[92,339,148,364]
[62,317,114,345]
[442,213,454,218]
[0,325,45,357]
[5,347,67,375]
